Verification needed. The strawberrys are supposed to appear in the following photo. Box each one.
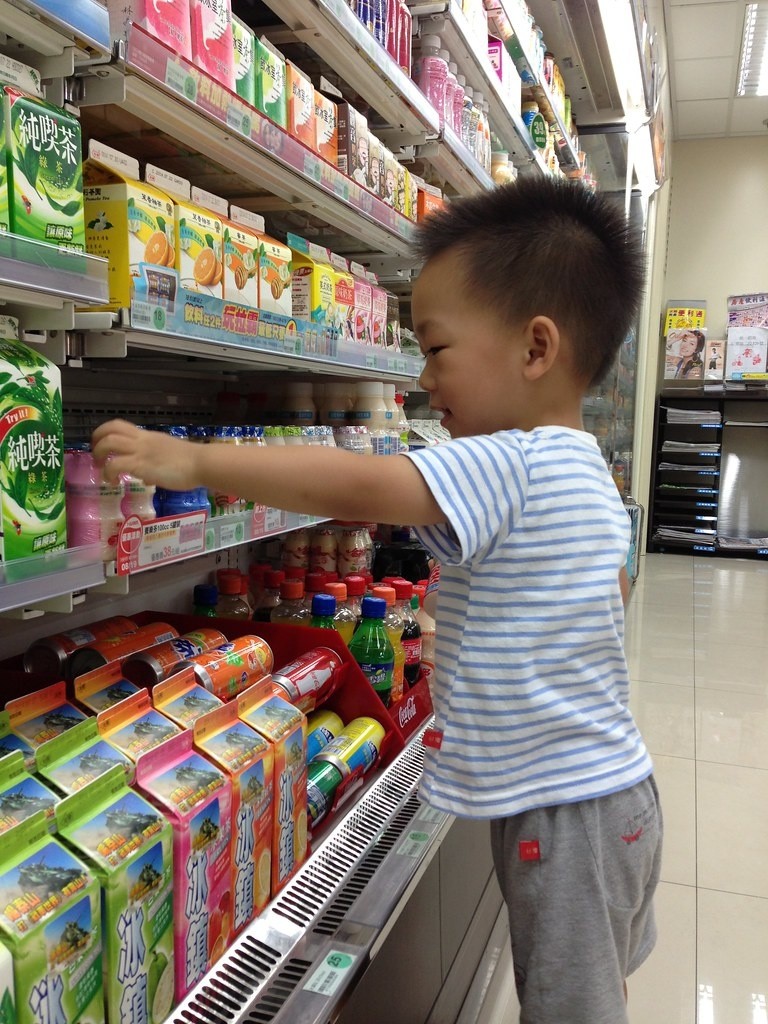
[356,316,380,344]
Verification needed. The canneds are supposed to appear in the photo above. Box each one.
[22,612,385,832]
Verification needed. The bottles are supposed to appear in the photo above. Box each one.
[613,464,624,497]
[423,34,518,186]
[60,376,437,708]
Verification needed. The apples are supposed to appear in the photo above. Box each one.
[146,950,174,1024]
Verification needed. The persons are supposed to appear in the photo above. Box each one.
[707,348,722,369]
[96,178,662,1024]
[666,329,704,380]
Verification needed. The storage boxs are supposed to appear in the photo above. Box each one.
[646,289,768,553]
[486,29,522,117]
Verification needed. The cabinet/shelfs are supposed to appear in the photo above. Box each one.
[0,0,661,1024]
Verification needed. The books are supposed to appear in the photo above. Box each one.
[666,330,705,381]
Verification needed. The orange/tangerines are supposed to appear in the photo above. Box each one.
[142,231,223,286]
